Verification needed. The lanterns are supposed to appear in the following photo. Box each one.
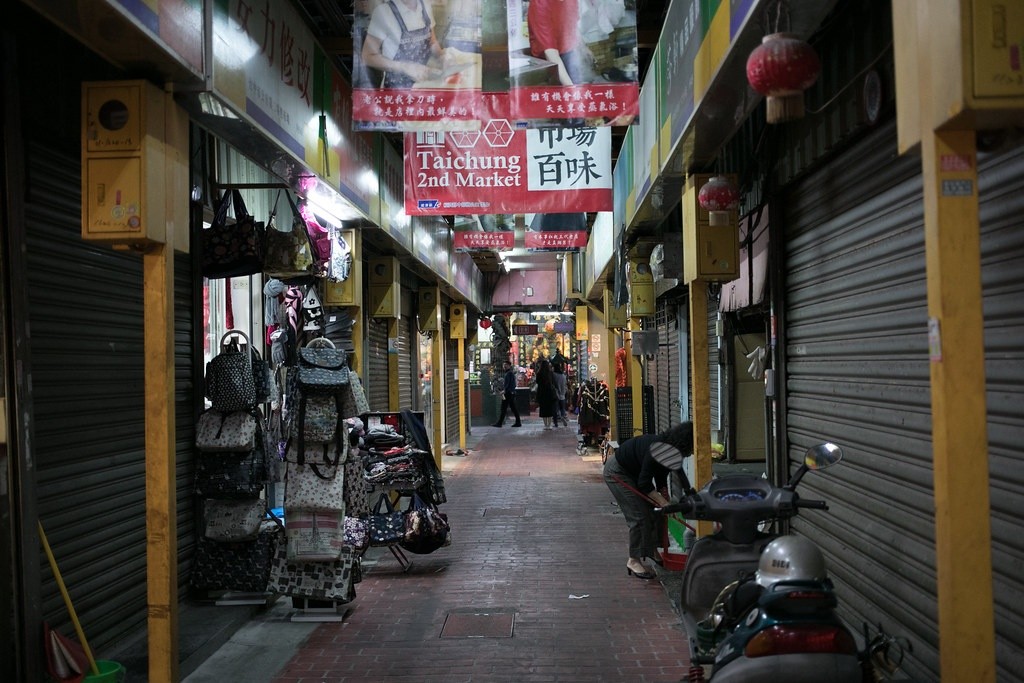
[698,177,741,227]
[480,318,491,329]
[545,319,556,336]
[747,31,821,124]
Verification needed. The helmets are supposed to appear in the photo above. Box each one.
[756,535,824,592]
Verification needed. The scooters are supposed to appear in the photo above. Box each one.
[648,438,879,683]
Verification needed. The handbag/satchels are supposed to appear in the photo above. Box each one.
[304,218,332,267]
[267,342,371,604]
[201,189,265,280]
[398,492,451,554]
[369,492,405,547]
[423,467,447,505]
[185,339,285,593]
[549,382,565,400]
[262,188,322,281]
[342,515,366,548]
[328,231,352,283]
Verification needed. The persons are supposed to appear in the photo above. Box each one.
[528,0,581,86]
[552,363,568,427]
[536,360,558,429]
[488,360,522,428]
[530,352,545,373]
[549,348,564,364]
[603,419,694,580]
[362,0,442,87]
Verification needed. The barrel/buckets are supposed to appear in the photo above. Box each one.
[82,660,122,683]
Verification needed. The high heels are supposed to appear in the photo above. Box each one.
[626,559,653,579]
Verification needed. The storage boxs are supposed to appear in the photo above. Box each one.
[615,385,655,444]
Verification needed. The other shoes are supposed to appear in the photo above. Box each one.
[544,426,549,430]
[548,425,554,431]
[511,423,522,427]
[491,422,503,427]
[561,417,568,427]
